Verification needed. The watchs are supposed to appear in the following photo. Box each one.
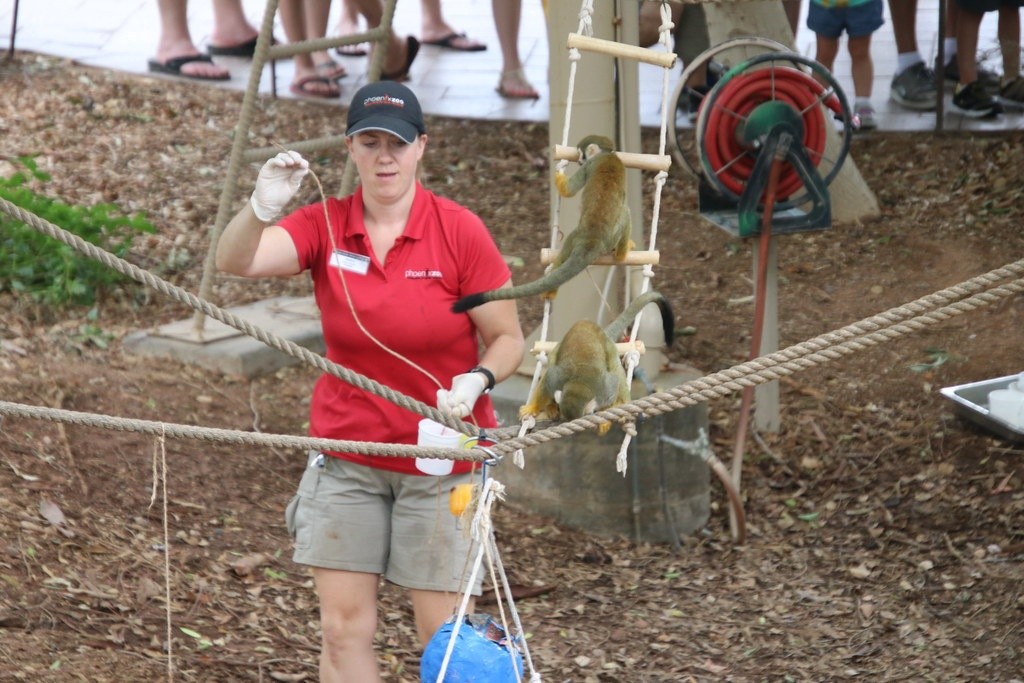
[471,366,495,395]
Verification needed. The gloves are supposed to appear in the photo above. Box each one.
[436,372,484,418]
[250,149,309,221]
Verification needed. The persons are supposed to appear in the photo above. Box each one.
[215,80,527,683]
[278,1,540,100]
[147,1,296,79]
[888,0,1024,120]
[806,0,884,128]
[614,0,800,123]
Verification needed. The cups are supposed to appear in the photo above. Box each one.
[414,418,464,476]
[988,388,1024,429]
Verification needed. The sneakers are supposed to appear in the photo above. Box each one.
[991,76,1023,110]
[933,53,1002,95]
[890,61,941,111]
[951,82,1003,118]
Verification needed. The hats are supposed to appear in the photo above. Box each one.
[345,80,426,144]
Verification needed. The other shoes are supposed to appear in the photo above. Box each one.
[685,87,715,118]
[852,102,877,128]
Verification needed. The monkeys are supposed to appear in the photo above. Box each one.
[451,134,637,314]
[515,319,629,437]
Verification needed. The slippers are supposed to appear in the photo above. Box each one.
[495,79,539,100]
[290,75,341,98]
[418,32,487,52]
[209,32,281,57]
[149,53,231,82]
[336,43,368,56]
[380,36,421,81]
[315,59,346,78]
